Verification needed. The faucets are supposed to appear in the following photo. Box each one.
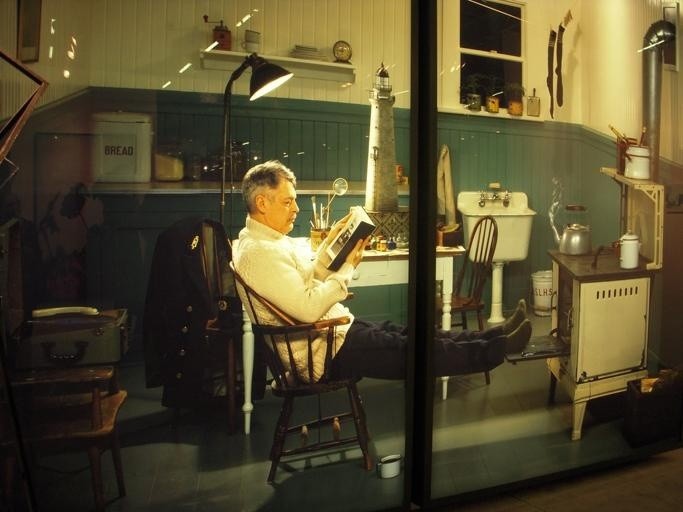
[492,193,499,200]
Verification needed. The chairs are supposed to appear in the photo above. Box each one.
[436,216,499,385]
[171,220,256,432]
[229,260,374,484]
[333,41,354,65]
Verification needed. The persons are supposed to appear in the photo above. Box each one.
[231,160,533,381]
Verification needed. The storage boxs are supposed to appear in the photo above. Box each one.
[82,110,154,185]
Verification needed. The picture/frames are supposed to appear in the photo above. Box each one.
[16,0,42,62]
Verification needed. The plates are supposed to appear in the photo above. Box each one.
[288,45,327,60]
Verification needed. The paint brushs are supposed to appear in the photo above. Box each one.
[309,192,337,230]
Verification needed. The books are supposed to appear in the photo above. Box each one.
[324,204,376,273]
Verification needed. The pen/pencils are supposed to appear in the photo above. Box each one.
[609,125,647,147]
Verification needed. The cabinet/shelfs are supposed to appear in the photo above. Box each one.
[81,48,411,196]
[0,364,127,512]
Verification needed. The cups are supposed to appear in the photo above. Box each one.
[244,29,261,44]
[375,453,402,480]
[241,41,259,53]
[309,226,331,252]
[616,137,646,176]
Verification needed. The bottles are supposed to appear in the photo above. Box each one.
[371,236,397,253]
[624,146,650,179]
[186,152,202,181]
[154,143,184,181]
[619,229,639,269]
[212,20,232,51]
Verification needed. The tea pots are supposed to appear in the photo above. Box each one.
[551,205,593,255]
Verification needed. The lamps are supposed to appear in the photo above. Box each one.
[220,52,295,226]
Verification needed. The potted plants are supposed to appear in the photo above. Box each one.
[460,75,525,118]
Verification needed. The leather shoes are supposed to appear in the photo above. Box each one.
[504,299,526,334]
[506,319,532,354]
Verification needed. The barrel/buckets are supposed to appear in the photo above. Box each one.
[529,269,553,317]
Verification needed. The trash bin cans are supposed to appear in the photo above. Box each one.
[621,374,683,449]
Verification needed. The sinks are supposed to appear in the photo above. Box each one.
[457,192,538,263]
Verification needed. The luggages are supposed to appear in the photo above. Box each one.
[0,217,129,368]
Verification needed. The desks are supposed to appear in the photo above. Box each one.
[229,237,467,436]
